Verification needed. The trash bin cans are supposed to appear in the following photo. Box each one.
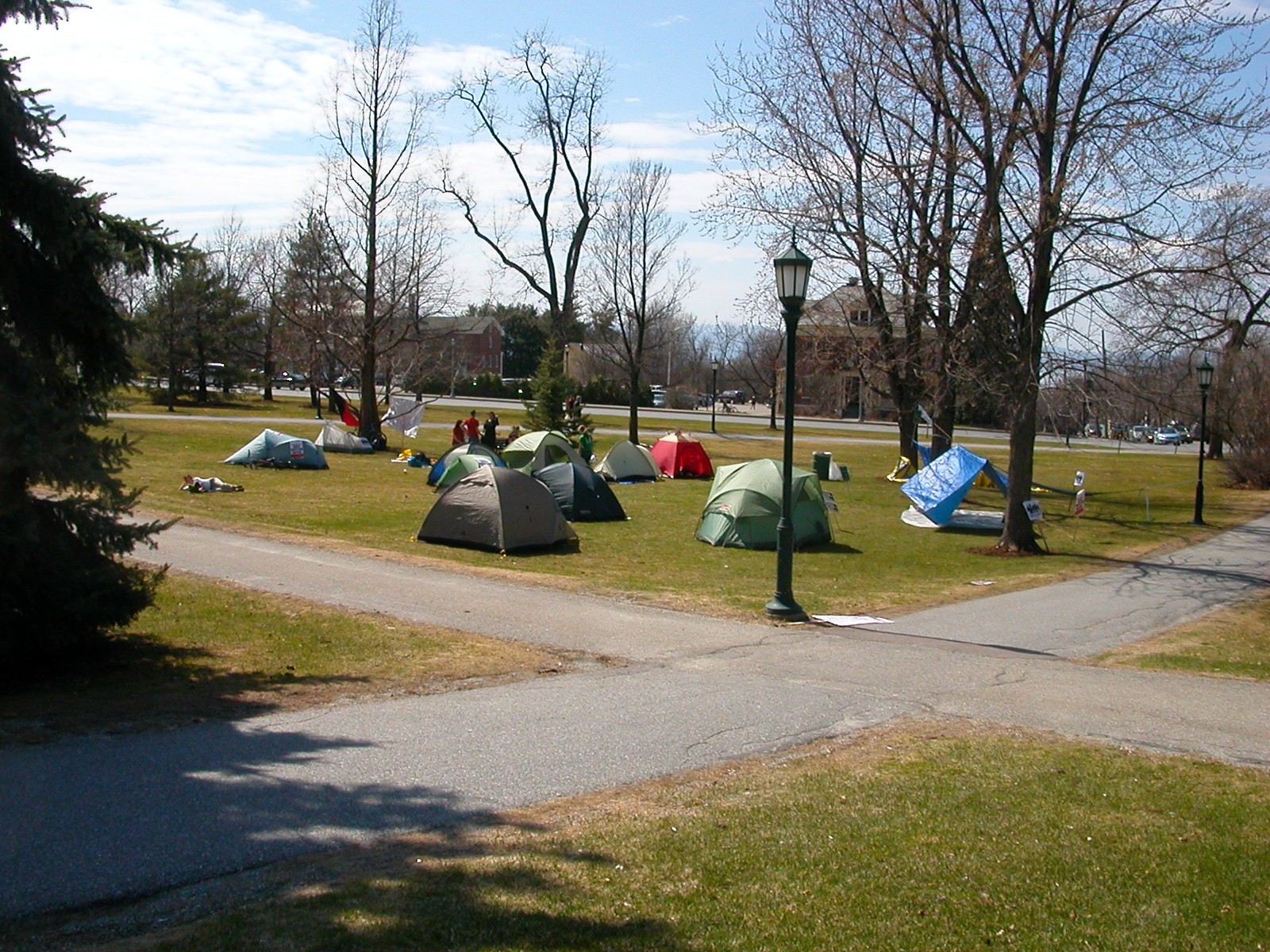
[812,451,832,481]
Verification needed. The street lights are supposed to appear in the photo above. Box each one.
[762,225,814,617]
[710,356,719,433]
[1187,353,1216,526]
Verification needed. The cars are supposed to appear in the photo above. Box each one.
[374,371,403,388]
[249,367,362,390]
[1084,419,1209,446]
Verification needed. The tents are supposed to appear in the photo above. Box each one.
[426,441,504,486]
[899,444,1008,525]
[434,454,496,493]
[501,431,588,476]
[650,430,714,479]
[533,461,628,523]
[593,439,662,484]
[417,464,578,557]
[694,458,832,549]
[223,428,329,470]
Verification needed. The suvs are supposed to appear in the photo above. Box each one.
[649,384,747,409]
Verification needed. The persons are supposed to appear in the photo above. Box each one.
[578,425,592,464]
[564,395,581,433]
[750,396,756,410]
[460,410,480,444]
[508,426,519,443]
[452,420,464,446]
[482,411,498,449]
[179,474,244,492]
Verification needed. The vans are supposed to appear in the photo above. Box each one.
[181,362,227,388]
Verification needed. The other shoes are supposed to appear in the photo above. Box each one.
[237,485,242,487]
[237,488,244,491]
